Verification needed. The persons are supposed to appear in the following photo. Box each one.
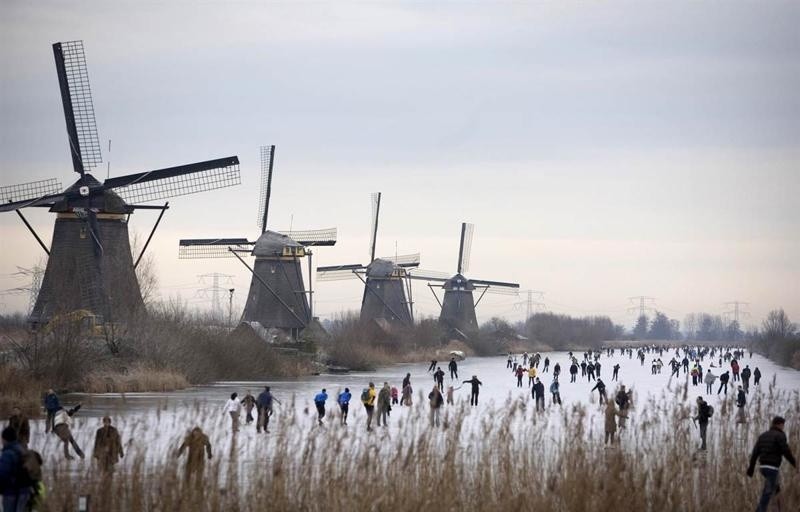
[92,416,124,479]
[176,426,212,490]
[746,415,795,511]
[53,400,86,460]
[0,425,32,512]
[43,388,59,434]
[504,342,762,454]
[9,406,30,450]
[219,357,483,434]
